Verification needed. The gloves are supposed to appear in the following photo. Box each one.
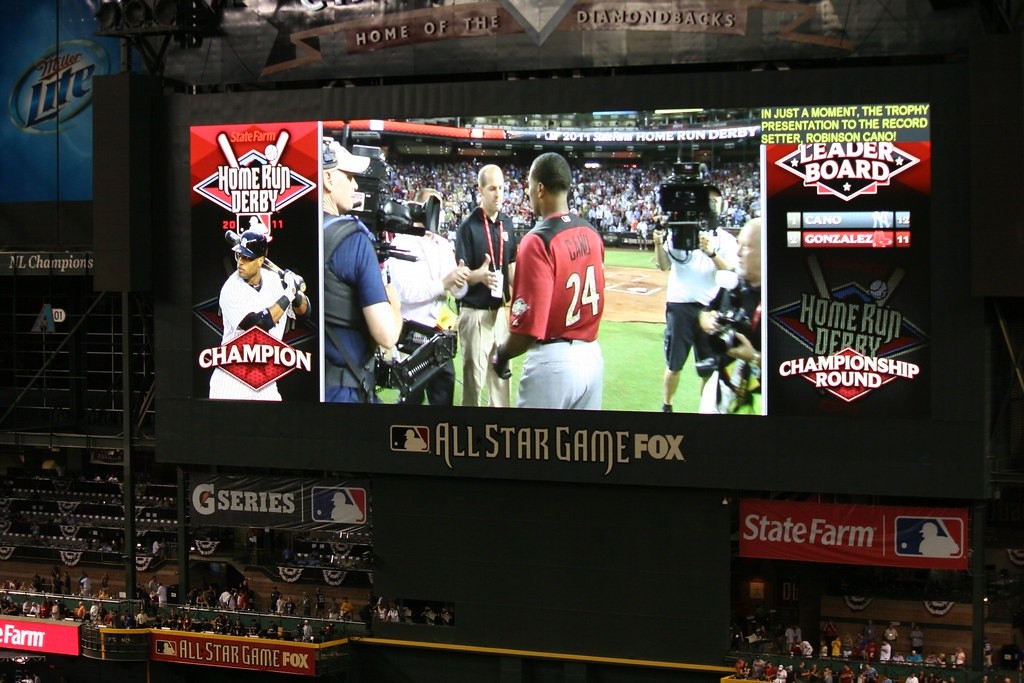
[283,271,303,300]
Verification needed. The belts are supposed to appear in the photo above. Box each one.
[533,335,578,345]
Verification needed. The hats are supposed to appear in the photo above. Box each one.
[230,230,268,260]
[703,181,723,197]
[322,134,370,173]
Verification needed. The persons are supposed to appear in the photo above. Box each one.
[0,467,454,645]
[490,152,607,411]
[646,182,743,413]
[699,218,762,420]
[207,224,313,403]
[379,188,468,406]
[374,114,762,250]
[730,611,1024,682]
[322,136,398,405]
[453,164,518,408]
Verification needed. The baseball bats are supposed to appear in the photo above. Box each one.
[225,231,307,292]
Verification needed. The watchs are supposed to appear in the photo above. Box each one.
[707,248,717,258]
[749,352,760,369]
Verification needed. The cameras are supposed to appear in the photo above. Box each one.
[707,314,752,355]
